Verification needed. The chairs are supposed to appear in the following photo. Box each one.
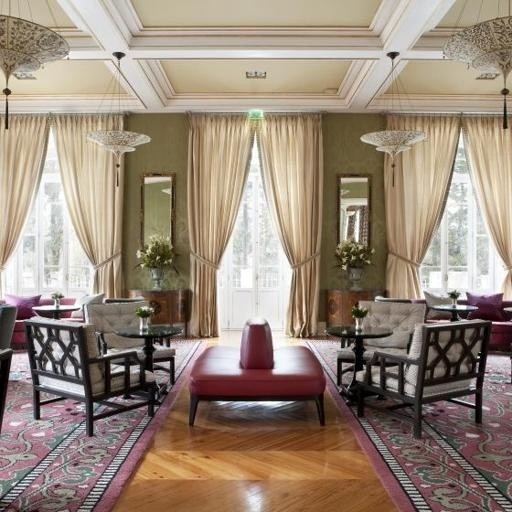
[82,302,176,385]
[336,299,428,404]
[24,315,157,437]
[0,301,20,436]
[354,318,493,437]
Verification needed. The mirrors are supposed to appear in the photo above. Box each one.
[336,173,371,263]
[137,171,177,258]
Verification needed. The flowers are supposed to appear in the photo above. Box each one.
[445,287,461,299]
[134,233,182,277]
[350,304,368,317]
[133,304,154,318]
[50,288,65,299]
[333,231,378,271]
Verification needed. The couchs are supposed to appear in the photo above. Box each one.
[0,292,143,351]
[373,295,512,352]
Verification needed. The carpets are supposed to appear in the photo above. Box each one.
[0,337,206,512]
[298,336,512,512]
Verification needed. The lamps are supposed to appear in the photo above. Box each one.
[441,1,512,133]
[357,49,431,188]
[0,0,72,129]
[85,53,153,186]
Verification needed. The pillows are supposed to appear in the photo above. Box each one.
[464,291,504,321]
[4,293,43,320]
[423,289,463,320]
[71,293,104,318]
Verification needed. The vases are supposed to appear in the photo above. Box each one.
[347,262,368,291]
[354,316,363,330]
[143,261,171,292]
[451,296,457,307]
[139,314,148,330]
[55,298,60,307]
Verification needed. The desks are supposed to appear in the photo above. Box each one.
[33,305,81,319]
[429,303,479,320]
[114,325,185,397]
[325,325,394,406]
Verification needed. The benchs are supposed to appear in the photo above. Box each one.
[186,316,327,428]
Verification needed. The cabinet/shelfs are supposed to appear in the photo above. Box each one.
[325,288,388,337]
[123,288,192,339]
